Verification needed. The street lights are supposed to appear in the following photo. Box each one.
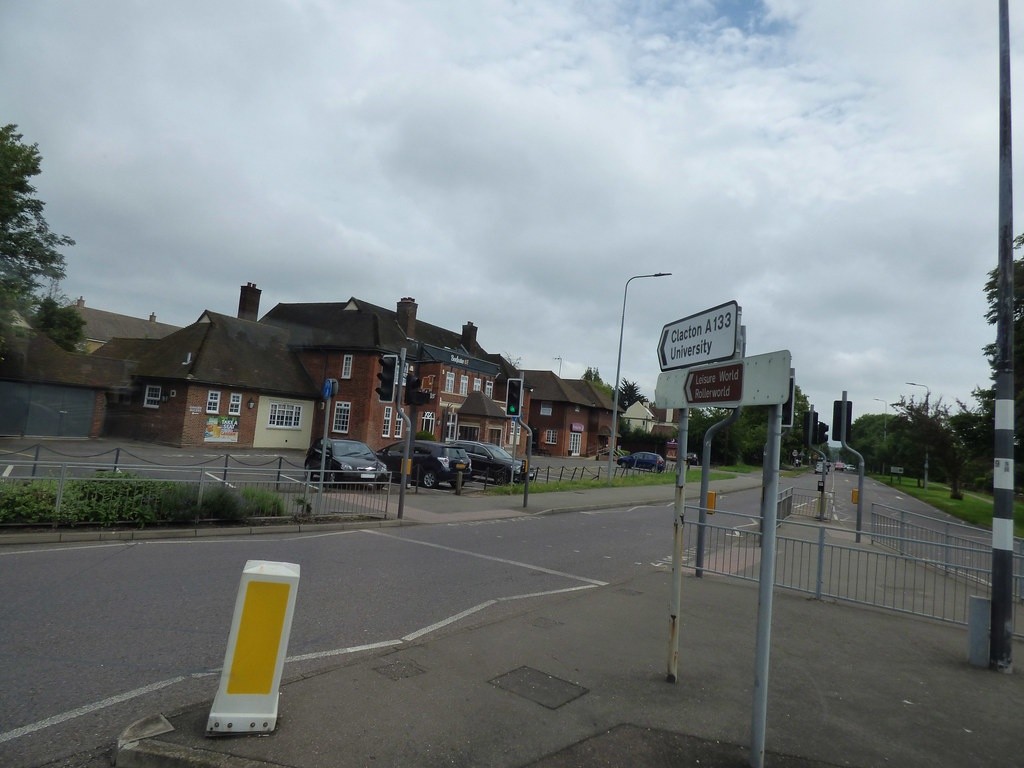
[607,273,673,483]
[906,382,929,489]
[875,398,887,475]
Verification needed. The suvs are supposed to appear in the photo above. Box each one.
[454,440,534,485]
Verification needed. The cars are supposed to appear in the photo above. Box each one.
[815,459,855,475]
[687,453,699,466]
[376,440,472,489]
[305,437,387,489]
[617,451,665,472]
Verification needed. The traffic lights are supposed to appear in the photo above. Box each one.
[818,421,829,444]
[505,378,523,418]
[375,355,398,403]
[405,373,430,405]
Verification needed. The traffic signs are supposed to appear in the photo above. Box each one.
[682,360,744,406]
[657,299,737,372]
